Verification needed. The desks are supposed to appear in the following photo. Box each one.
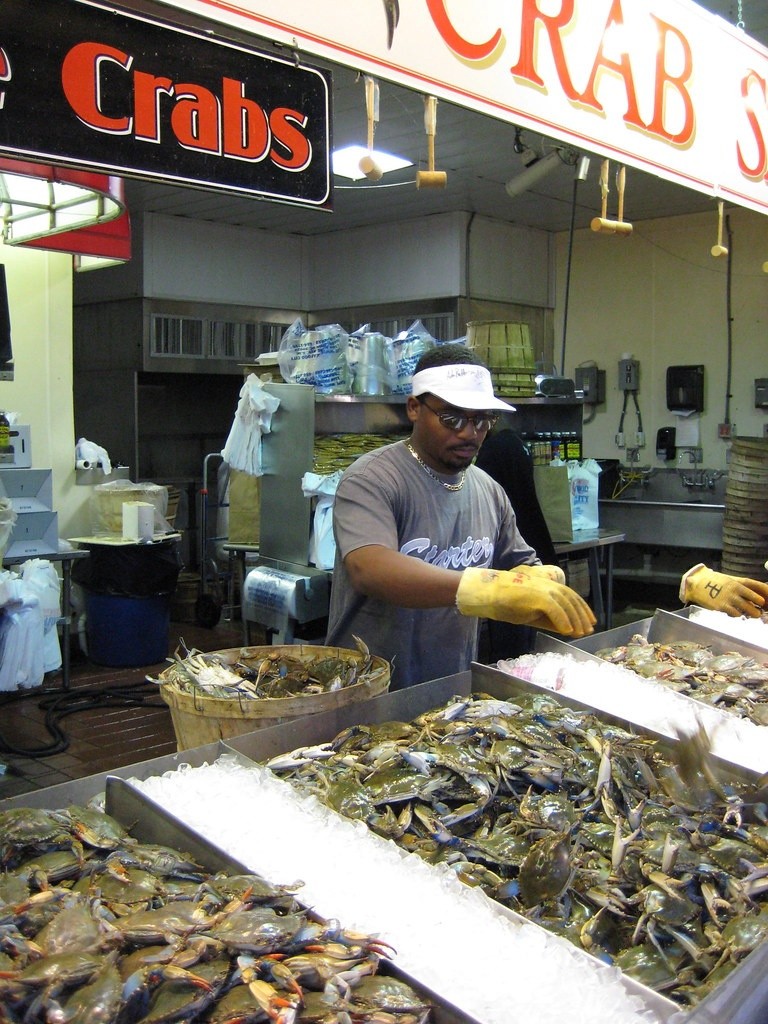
[2,548,91,688]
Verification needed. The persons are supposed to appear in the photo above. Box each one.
[324,344,597,690]
[475,429,560,664]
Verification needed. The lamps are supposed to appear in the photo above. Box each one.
[613,163,633,234]
[359,73,383,183]
[589,156,618,234]
[710,199,728,259]
[415,95,447,190]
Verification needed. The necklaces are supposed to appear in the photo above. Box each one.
[405,438,467,491]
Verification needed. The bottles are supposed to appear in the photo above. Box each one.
[520,430,582,466]
[0,411,10,449]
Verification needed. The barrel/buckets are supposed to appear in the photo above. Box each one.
[465,318,537,398]
[78,537,177,669]
[143,644,391,753]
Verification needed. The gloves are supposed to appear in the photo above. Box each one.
[508,565,565,586]
[679,563,768,618]
[456,567,597,638]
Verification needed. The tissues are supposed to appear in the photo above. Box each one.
[0,412,33,468]
[665,364,705,447]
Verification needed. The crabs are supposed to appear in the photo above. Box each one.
[144,633,373,699]
[595,634,768,727]
[0,802,440,1024]
[261,691,768,1008]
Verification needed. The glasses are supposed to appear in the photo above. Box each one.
[419,400,500,432]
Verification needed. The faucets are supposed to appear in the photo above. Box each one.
[678,451,697,485]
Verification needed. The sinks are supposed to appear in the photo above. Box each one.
[600,483,726,550]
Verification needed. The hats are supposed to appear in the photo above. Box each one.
[411,363,516,412]
